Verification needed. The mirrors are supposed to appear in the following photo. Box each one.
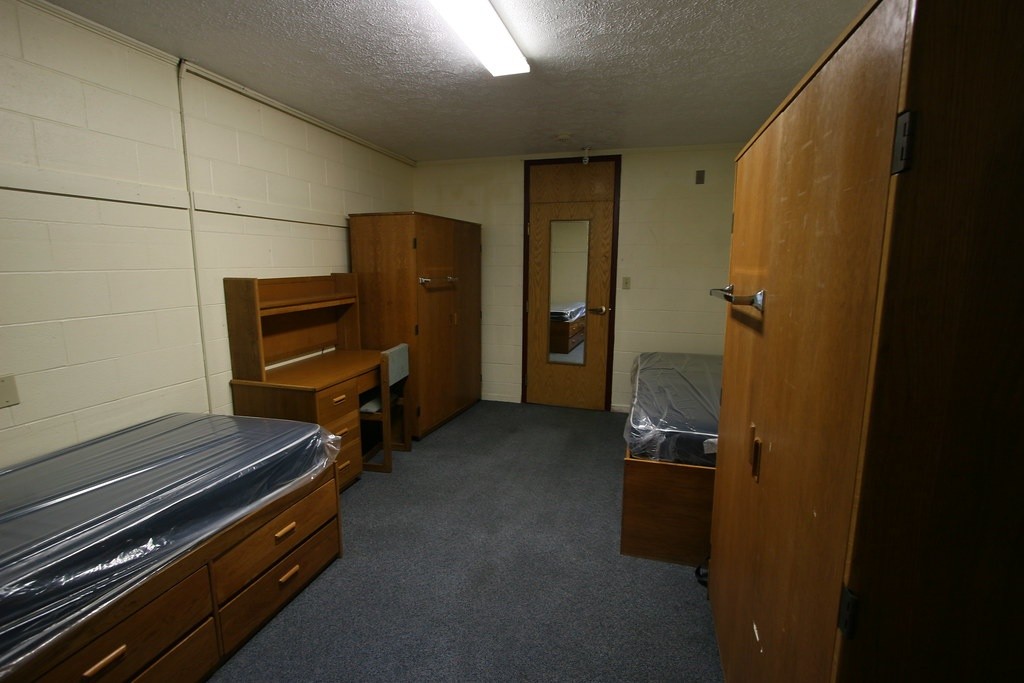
[548,220,590,365]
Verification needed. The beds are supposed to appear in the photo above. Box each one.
[620,353,724,568]
[0,411,343,683]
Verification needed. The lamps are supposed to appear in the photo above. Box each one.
[428,0,530,77]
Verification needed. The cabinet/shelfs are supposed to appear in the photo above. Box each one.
[550,301,585,354]
[709,0,1024,683]
[223,272,391,494]
[347,211,481,441]
